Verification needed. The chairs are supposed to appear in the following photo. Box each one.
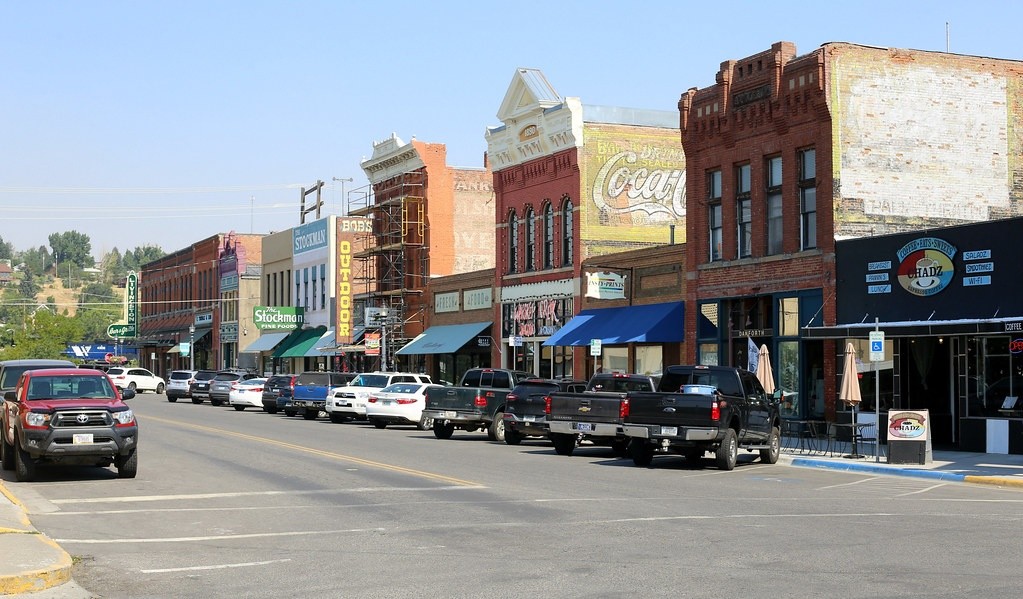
[78,381,96,396]
[32,381,50,398]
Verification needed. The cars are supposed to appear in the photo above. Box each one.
[366,381,445,431]
[229,377,269,411]
[102,366,166,395]
[0,359,84,402]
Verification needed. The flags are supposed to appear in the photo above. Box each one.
[747,336,760,373]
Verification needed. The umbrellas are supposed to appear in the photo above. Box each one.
[839,342,861,454]
[756,344,775,400]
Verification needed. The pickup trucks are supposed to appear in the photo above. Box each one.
[423,365,539,442]
[0,368,139,482]
[542,370,661,459]
[616,363,782,471]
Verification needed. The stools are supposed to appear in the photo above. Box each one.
[857,438,876,457]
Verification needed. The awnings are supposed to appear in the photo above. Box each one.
[541,301,685,346]
[304,328,365,356]
[243,332,290,351]
[166,329,211,352]
[700,312,718,339]
[395,321,494,354]
[270,327,326,357]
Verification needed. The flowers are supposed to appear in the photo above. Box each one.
[109,355,123,364]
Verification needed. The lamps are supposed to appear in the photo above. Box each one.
[244,328,246,335]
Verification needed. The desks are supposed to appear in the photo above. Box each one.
[831,423,874,457]
[780,420,829,455]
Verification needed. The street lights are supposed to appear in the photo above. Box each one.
[377,300,389,373]
[188,322,196,371]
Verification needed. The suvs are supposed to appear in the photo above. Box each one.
[325,370,434,425]
[262,373,298,414]
[165,370,198,402]
[208,369,263,407]
[189,369,223,404]
[285,370,360,421]
[501,376,589,447]
